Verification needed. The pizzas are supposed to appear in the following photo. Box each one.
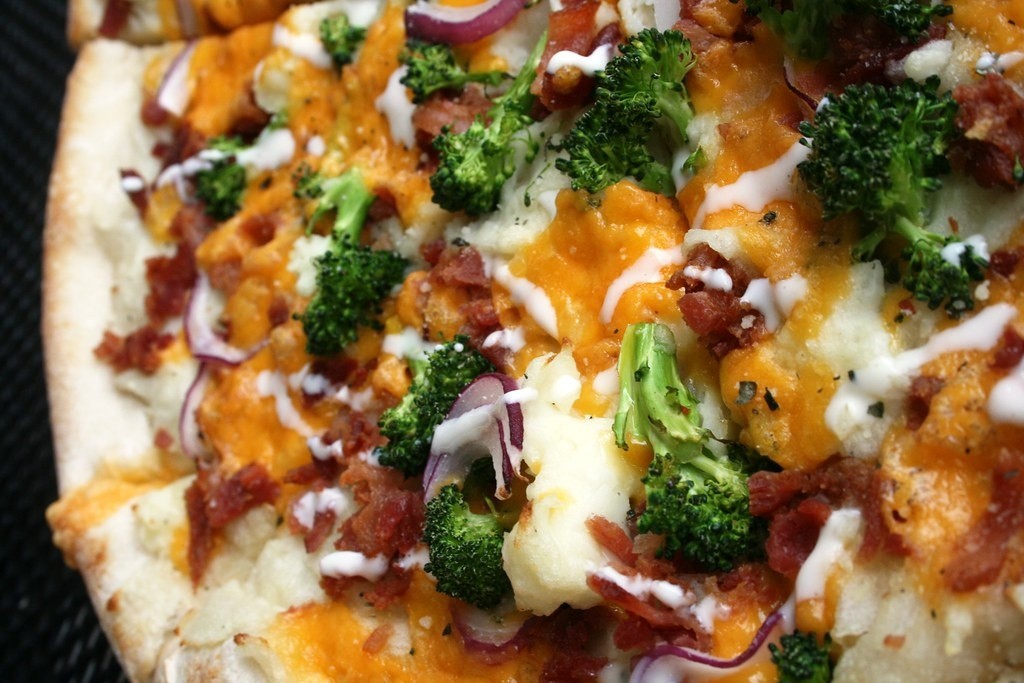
[39,0,1024,683]
